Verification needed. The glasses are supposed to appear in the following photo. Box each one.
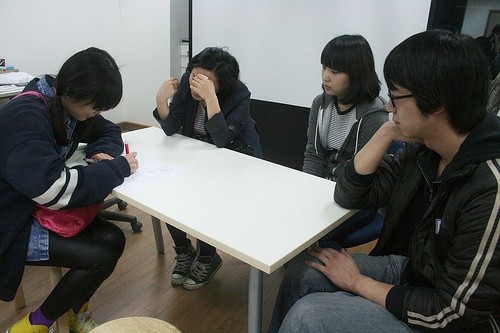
[387,93,415,107]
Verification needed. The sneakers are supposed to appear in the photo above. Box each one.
[169,239,196,285]
[182,251,223,290]
[67,311,98,333]
[6,311,55,333]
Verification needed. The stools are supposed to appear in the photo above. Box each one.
[86,315,182,333]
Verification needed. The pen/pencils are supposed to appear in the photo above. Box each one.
[125,140,129,154]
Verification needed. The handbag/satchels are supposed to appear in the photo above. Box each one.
[32,203,104,238]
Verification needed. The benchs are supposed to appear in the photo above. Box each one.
[249,98,312,172]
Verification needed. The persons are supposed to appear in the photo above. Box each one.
[303,34,406,185]
[267,29,500,333]
[0,48,129,333]
[474,25,500,81]
[153,47,264,290]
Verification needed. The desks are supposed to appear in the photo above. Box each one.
[0,84,25,100]
[65,126,358,333]
